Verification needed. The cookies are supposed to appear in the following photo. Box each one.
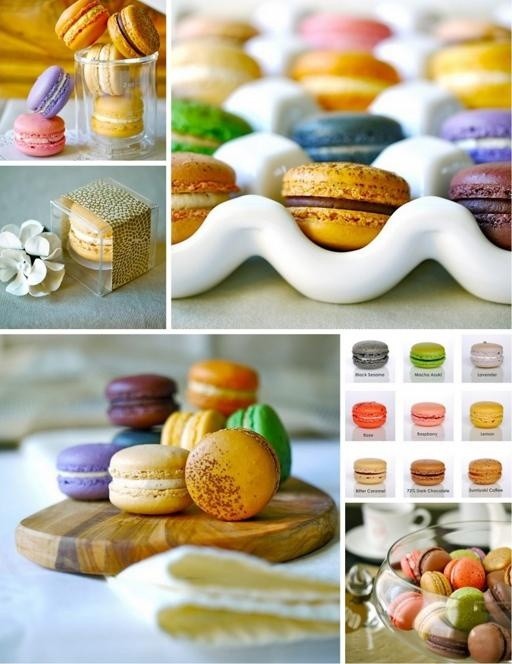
[352,402,504,428]
[386,546,512,663]
[429,40,511,111]
[281,160,408,250]
[172,100,252,160]
[172,14,256,44]
[290,116,402,165]
[433,17,501,42]
[443,112,511,163]
[448,161,512,252]
[55,359,291,521]
[13,0,160,157]
[298,12,391,50]
[353,459,501,486]
[171,152,234,245]
[68,202,113,262]
[352,341,503,369]
[171,42,261,106]
[289,49,401,114]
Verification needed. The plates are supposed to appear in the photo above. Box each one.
[346,525,433,562]
[437,511,495,529]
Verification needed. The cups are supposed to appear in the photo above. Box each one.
[72,49,159,161]
[362,502,433,548]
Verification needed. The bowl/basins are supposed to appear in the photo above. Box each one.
[371,518,512,664]
